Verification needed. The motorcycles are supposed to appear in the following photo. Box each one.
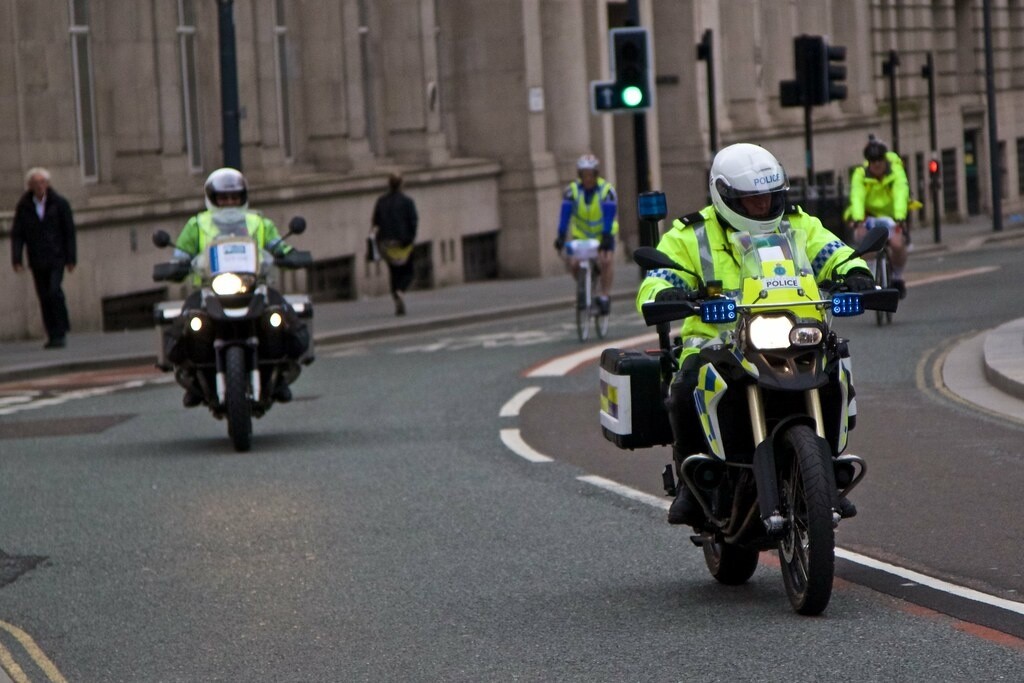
[152,216,316,453]
[599,224,899,617]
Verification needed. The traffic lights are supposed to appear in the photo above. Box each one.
[590,26,656,115]
[795,33,848,105]
[929,157,942,180]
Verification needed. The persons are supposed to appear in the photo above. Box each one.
[171,169,308,407]
[10,169,78,349]
[637,144,874,523]
[554,154,618,316]
[845,142,908,300]
[368,174,417,316]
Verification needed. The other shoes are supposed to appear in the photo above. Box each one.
[277,385,292,404]
[394,304,403,315]
[892,278,906,299]
[597,298,610,314]
[42,338,65,349]
[667,487,704,524]
[834,490,857,519]
[182,382,201,408]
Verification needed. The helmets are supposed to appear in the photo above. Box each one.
[575,154,599,169]
[708,142,789,234]
[204,168,249,226]
[861,140,887,156]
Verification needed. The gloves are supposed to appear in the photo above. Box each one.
[655,288,688,305]
[553,238,564,250]
[280,251,307,270]
[844,274,874,297]
[171,261,189,282]
[598,241,615,250]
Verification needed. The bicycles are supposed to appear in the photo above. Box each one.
[864,225,893,327]
[560,238,610,343]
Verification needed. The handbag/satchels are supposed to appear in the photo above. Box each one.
[364,231,382,262]
